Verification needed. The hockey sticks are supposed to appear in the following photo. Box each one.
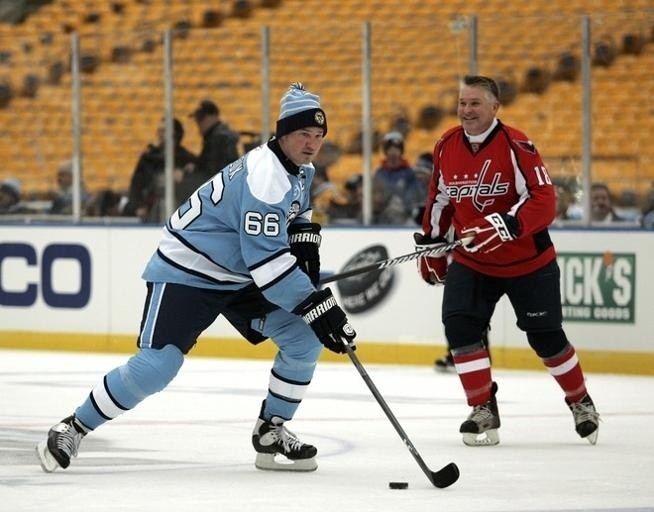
[343,336,459,488]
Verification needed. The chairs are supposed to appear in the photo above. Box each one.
[0,1,654,228]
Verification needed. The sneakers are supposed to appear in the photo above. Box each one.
[250,400,318,460]
[565,394,600,439]
[47,413,87,471]
[460,381,502,435]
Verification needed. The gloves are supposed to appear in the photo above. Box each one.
[288,220,326,289]
[289,286,359,355]
[411,230,448,286]
[459,213,520,255]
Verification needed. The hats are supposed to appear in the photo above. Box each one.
[414,153,433,177]
[381,131,405,156]
[188,100,218,119]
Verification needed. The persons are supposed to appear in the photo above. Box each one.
[38,76,359,470]
[51,164,119,217]
[0,178,24,214]
[412,75,600,446]
[128,100,238,223]
[553,182,654,230]
[309,130,433,223]
